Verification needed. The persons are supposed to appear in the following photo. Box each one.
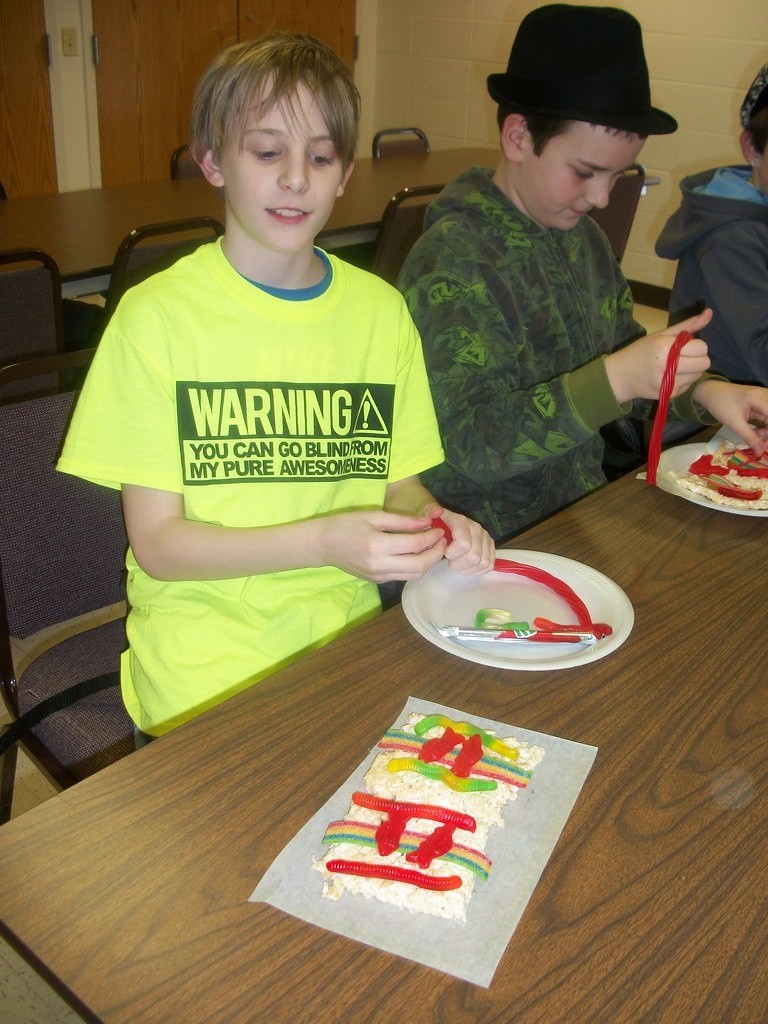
[58,27,496,752]
[396,4,767,543]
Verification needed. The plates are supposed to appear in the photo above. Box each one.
[656,443,768,517]
[402,550,636,672]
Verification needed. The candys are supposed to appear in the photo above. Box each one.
[430,515,611,644]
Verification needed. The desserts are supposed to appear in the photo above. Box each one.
[671,444,768,510]
[323,793,490,921]
[368,716,541,816]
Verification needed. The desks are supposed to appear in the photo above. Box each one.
[0,149,660,299]
[0,425,768,1024]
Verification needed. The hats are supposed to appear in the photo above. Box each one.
[487,3,678,135]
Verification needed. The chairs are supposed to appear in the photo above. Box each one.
[0,126,449,824]
